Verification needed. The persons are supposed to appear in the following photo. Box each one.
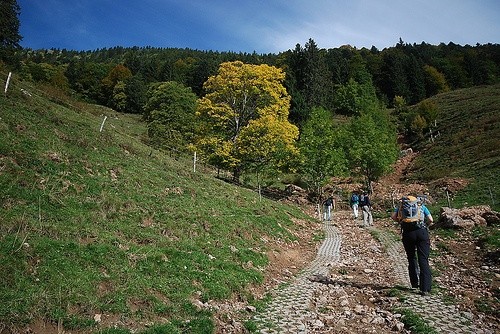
[321,195,336,220]
[360,191,374,228]
[350,193,360,219]
[391,193,433,296]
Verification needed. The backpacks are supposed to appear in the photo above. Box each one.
[398,195,422,223]
[351,194,359,202]
[326,198,332,206]
[359,194,365,204]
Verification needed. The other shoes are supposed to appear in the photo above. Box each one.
[412,280,418,288]
[328,219,332,221]
[323,219,325,220]
[364,224,368,227]
[354,217,358,219]
[422,290,429,295]
[369,223,373,226]
